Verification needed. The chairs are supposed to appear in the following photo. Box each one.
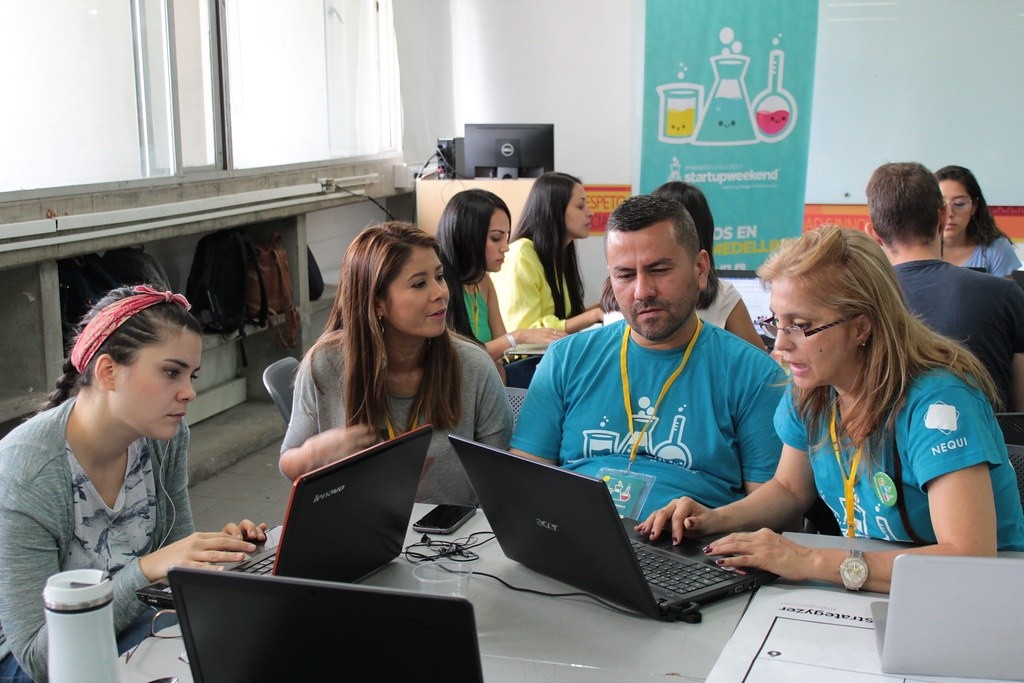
[994,413,1024,513]
[262,356,300,426]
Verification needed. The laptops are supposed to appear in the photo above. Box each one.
[166,566,484,683]
[870,554,1024,681]
[446,433,783,620]
[135,424,434,609]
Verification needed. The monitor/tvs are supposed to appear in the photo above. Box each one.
[463,124,554,180]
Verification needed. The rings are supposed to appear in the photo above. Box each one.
[554,331,557,336]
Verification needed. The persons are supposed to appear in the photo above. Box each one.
[279,222,515,507]
[435,189,569,365]
[0,285,267,683]
[933,165,1024,278]
[864,162,1024,441]
[598,181,766,351]
[633,224,1023,593]
[490,172,604,358]
[507,194,803,533]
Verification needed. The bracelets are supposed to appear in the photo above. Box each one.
[505,333,516,347]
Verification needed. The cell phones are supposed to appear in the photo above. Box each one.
[412,503,477,534]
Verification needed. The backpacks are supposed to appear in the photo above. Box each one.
[186,226,300,352]
[56,244,172,359]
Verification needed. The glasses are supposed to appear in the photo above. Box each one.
[943,197,977,213]
[759,313,862,343]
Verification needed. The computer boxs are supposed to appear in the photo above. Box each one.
[435,136,465,180]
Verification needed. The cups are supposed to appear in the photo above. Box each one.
[43,572,122,683]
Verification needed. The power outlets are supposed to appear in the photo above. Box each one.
[393,162,424,189]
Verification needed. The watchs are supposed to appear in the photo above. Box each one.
[839,549,869,592]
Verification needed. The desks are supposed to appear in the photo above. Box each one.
[119,503,1024,683]
[415,172,537,242]
